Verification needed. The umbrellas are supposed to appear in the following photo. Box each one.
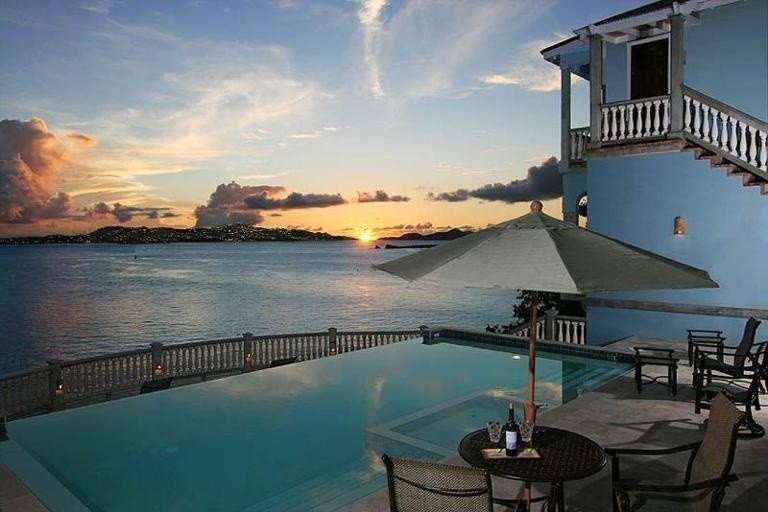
[370,201,722,511]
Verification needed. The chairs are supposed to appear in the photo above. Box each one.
[692,317,768,438]
[605,394,749,511]
[382,453,520,511]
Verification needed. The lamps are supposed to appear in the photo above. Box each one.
[674,217,685,237]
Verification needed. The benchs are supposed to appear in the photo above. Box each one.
[140,377,173,394]
[267,356,298,369]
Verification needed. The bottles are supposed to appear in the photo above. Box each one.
[505,404,518,456]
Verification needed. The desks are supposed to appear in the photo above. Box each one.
[633,347,679,397]
[687,328,726,367]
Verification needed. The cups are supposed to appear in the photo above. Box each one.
[486,422,502,442]
[519,420,534,442]
